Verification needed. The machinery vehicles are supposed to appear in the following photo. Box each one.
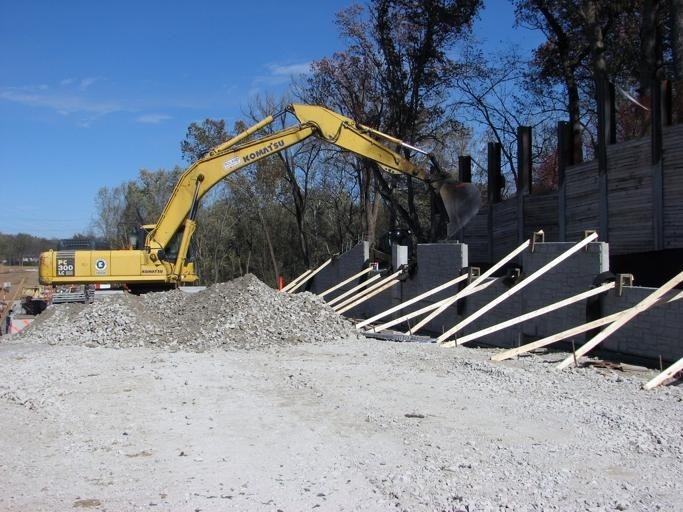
[37,104,483,303]
